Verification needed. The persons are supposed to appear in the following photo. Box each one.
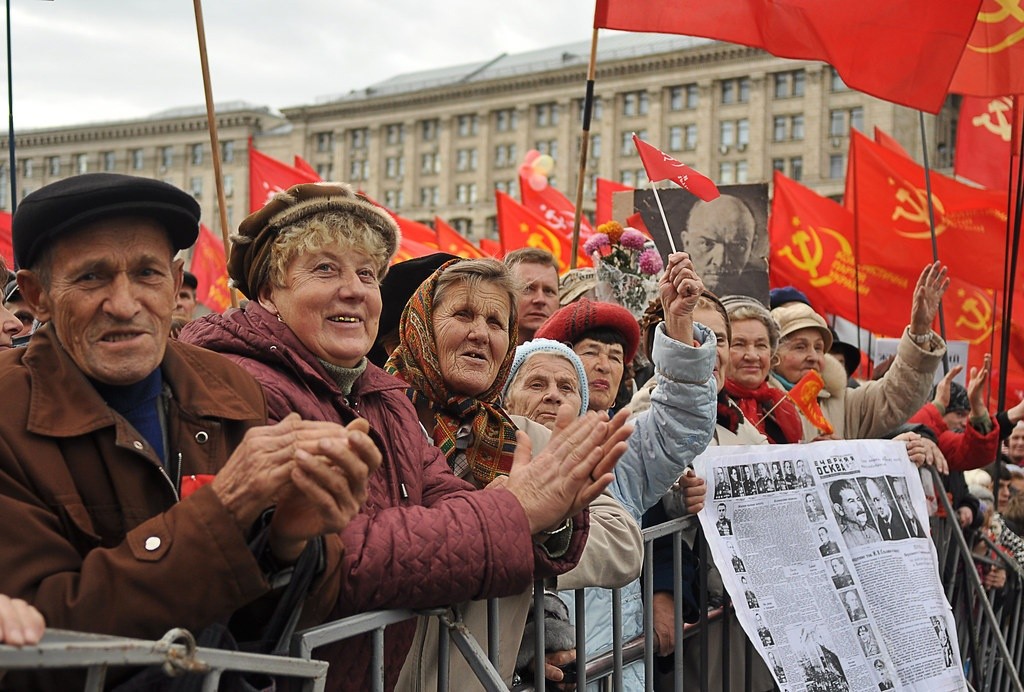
[831,558,854,589]
[676,193,759,296]
[796,460,816,488]
[771,462,787,491]
[858,625,879,657]
[714,467,731,499]
[818,527,840,557]
[846,591,866,622]
[716,503,733,537]
[770,258,950,446]
[741,465,757,496]
[936,620,953,667]
[741,576,760,608]
[366,259,644,692]
[0,172,386,692]
[171,185,628,692]
[805,494,827,521]
[829,479,882,549]
[866,478,910,541]
[892,478,927,538]
[0,252,1024,692]
[728,466,745,497]
[767,651,787,683]
[756,463,775,494]
[755,613,775,647]
[726,540,746,572]
[874,659,895,691]
[783,461,798,490]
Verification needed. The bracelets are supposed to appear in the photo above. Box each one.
[908,329,934,344]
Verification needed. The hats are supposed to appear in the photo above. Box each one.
[12,173,200,270]
[226,183,402,300]
[500,337,589,417]
[767,288,832,354]
[365,253,476,370]
[718,294,779,359]
[533,295,640,365]
[934,382,970,414]
[825,325,861,378]
[643,289,732,364]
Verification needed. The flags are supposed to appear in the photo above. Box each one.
[0,0,1024,434]
[786,365,838,436]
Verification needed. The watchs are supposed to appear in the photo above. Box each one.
[541,517,572,538]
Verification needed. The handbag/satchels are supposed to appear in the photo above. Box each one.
[122,527,325,692]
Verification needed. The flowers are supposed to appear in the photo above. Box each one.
[582,220,663,313]
